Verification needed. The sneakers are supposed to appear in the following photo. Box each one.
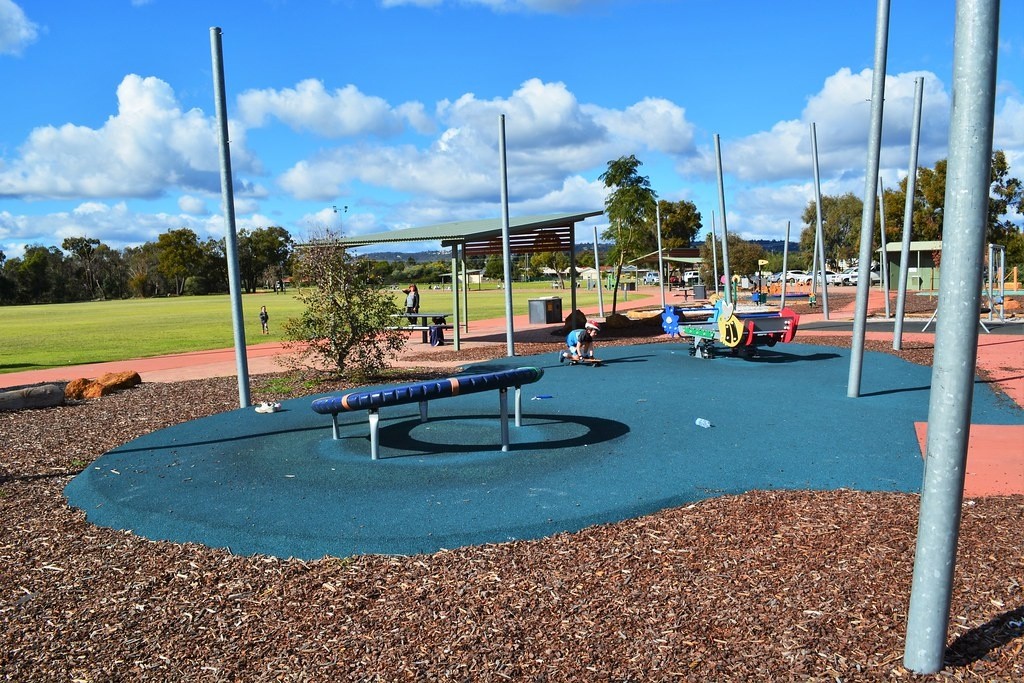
[262,401,281,411]
[255,402,274,413]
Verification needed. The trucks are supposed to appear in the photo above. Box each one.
[642,272,659,285]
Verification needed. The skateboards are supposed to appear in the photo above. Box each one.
[569,358,605,367]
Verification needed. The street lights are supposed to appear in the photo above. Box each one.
[334,205,348,239]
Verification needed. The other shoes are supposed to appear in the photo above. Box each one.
[559,350,565,363]
[582,354,590,358]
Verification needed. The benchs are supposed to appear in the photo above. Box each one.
[382,324,456,344]
[672,294,695,301]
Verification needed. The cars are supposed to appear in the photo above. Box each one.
[804,271,842,286]
[682,271,699,282]
[780,270,807,283]
[829,268,856,286]
[768,272,783,283]
[849,264,884,286]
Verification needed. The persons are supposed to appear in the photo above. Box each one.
[402,284,420,325]
[751,290,761,306]
[260,306,269,334]
[559,320,601,363]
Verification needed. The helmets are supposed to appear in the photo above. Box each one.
[585,319,600,331]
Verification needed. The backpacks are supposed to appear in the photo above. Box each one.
[430,327,444,346]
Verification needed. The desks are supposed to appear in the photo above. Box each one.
[677,286,691,300]
[389,313,453,345]
